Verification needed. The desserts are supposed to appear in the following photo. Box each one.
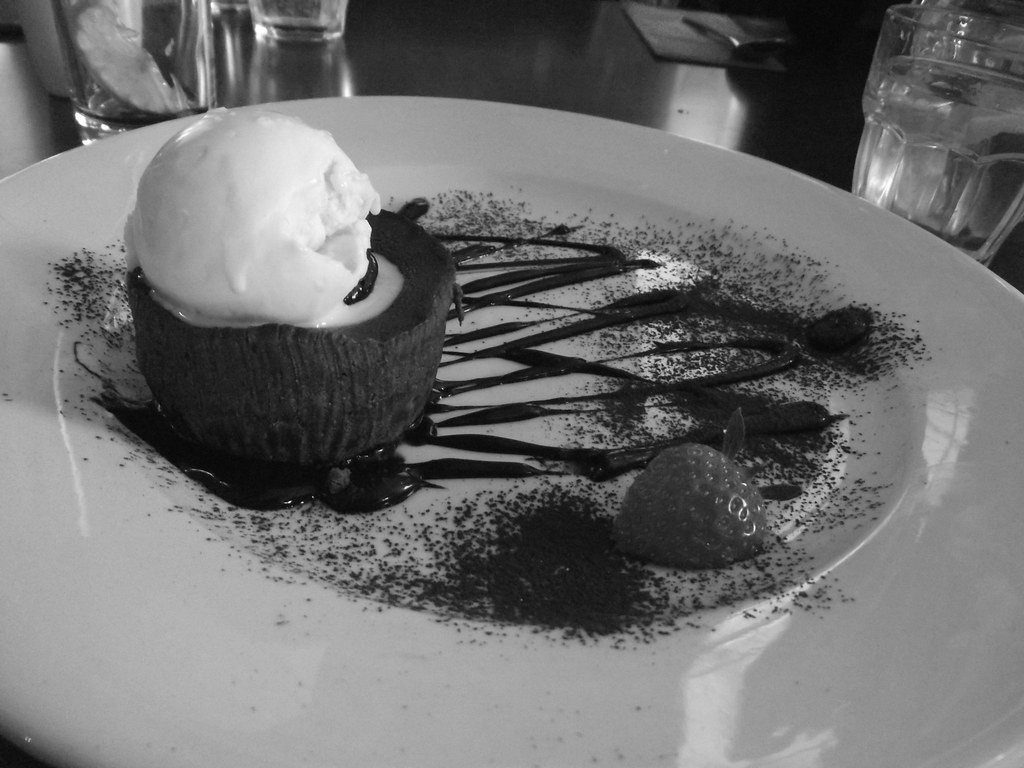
[122,106,455,470]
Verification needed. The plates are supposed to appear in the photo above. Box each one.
[0,95,1024,768]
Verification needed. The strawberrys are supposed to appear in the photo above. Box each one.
[612,405,803,568]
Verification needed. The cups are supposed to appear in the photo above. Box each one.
[248,0,347,45]
[852,5,1024,270]
[49,0,218,146]
